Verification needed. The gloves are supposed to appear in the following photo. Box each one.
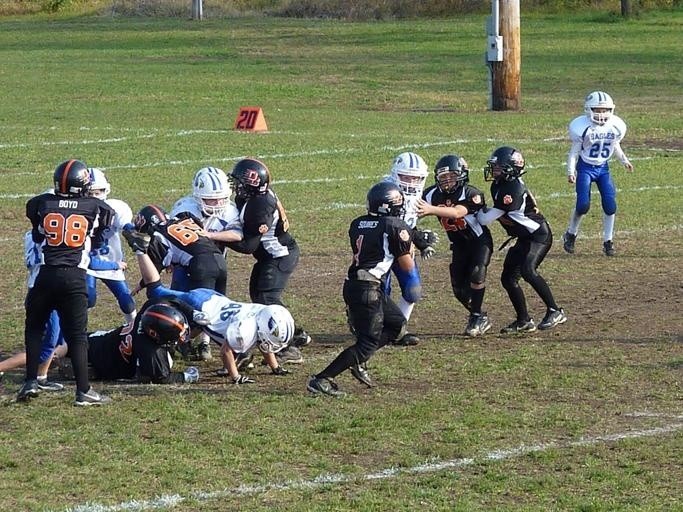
[273,367,293,376]
[420,246,436,261]
[231,376,258,384]
[415,230,440,244]
[183,366,200,383]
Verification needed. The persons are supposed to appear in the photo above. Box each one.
[416,155,493,338]
[122,228,294,384]
[564,91,634,256]
[169,167,243,361]
[223,158,312,366]
[16,159,116,406]
[86,168,136,327]
[307,182,414,398]
[0,189,67,392]
[346,152,439,345]
[66,294,209,384]
[131,205,254,377]
[476,147,567,335]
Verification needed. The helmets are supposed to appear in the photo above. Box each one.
[366,182,404,220]
[192,166,233,220]
[141,303,189,346]
[255,303,295,355]
[85,167,111,198]
[53,158,92,199]
[226,157,270,202]
[484,146,526,185]
[391,151,428,198]
[434,154,469,197]
[584,91,616,127]
[135,204,169,231]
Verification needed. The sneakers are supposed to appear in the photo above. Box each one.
[291,330,312,346]
[350,363,376,387]
[16,380,39,402]
[206,350,255,377]
[481,313,493,337]
[197,342,212,361]
[497,318,536,337]
[602,241,616,257]
[395,334,420,346]
[37,376,64,391]
[563,231,577,253]
[75,388,116,406]
[174,339,200,361]
[121,229,151,254]
[459,314,484,337]
[537,308,568,330]
[306,374,348,398]
[261,346,304,367]
